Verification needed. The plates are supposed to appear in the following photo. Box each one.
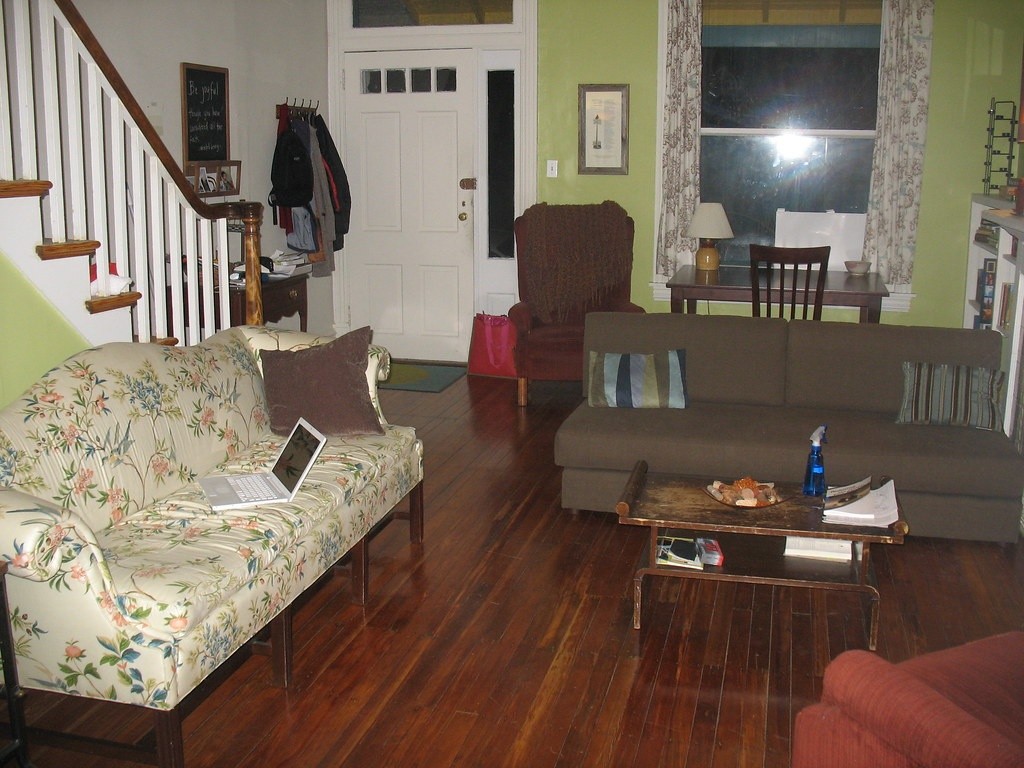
[701,482,794,509]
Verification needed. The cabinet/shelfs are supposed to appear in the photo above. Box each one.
[946,194,1023,436]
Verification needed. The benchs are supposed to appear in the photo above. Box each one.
[0,323,424,767]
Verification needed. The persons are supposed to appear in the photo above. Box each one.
[200,168,211,192]
[221,171,234,190]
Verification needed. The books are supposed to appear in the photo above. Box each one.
[656,536,704,570]
[974,219,1001,250]
[972,256,997,330]
[269,249,312,276]
[783,535,855,563]
[997,281,1015,329]
[823,476,875,519]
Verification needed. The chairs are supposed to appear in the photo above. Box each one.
[750,242,829,320]
[514,199,645,412]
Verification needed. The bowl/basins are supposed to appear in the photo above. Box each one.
[845,261,872,276]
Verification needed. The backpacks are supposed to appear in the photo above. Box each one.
[268,118,313,225]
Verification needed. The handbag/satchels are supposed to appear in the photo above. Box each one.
[468,313,518,379]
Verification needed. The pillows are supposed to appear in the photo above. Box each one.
[583,347,686,409]
[256,325,388,436]
[899,361,1005,430]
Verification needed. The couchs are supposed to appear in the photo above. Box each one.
[791,626,1024,768]
[555,315,1024,541]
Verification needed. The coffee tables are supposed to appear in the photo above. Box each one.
[617,456,909,650]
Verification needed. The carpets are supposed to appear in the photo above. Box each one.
[377,363,468,393]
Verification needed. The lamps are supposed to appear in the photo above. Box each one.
[683,202,734,272]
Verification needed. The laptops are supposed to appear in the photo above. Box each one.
[197,417,326,512]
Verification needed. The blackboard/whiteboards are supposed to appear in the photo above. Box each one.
[180,61,230,166]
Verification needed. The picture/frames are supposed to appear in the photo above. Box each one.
[577,83,632,176]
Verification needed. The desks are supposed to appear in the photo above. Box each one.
[166,272,311,339]
[667,262,890,324]
